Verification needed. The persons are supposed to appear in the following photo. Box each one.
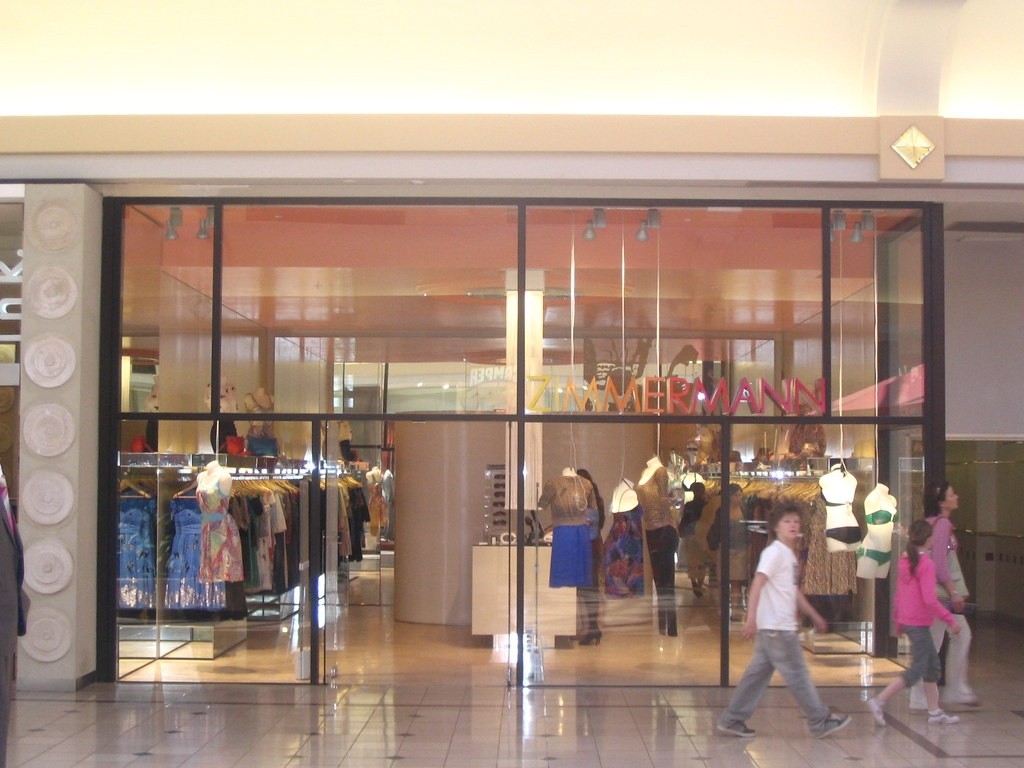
[636,457,677,636]
[144,374,159,451]
[678,482,711,596]
[680,470,705,504]
[856,483,897,579]
[819,463,861,552]
[195,460,245,583]
[909,480,983,709]
[366,467,394,540]
[539,467,599,590]
[867,519,961,724]
[577,469,605,645]
[0,464,32,768]
[715,483,751,621]
[716,503,852,739]
[604,478,644,598]
[205,375,238,453]
[789,402,827,456]
[244,387,274,437]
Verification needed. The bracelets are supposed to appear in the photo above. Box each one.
[950,591,958,596]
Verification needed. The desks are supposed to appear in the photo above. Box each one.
[472,546,577,649]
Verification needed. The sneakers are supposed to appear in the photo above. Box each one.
[927,708,960,725]
[946,693,983,711]
[717,719,756,737]
[868,697,887,727]
[818,713,851,739]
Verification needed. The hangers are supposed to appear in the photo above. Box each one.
[701,477,822,505]
[173,473,368,499]
[119,479,152,499]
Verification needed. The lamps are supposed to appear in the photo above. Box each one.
[594,207,606,228]
[830,208,874,243]
[197,218,209,239]
[648,209,660,228]
[637,220,648,240]
[165,220,178,240]
[584,220,595,240]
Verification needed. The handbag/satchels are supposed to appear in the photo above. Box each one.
[933,548,969,600]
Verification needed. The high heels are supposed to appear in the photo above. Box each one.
[579,630,602,646]
